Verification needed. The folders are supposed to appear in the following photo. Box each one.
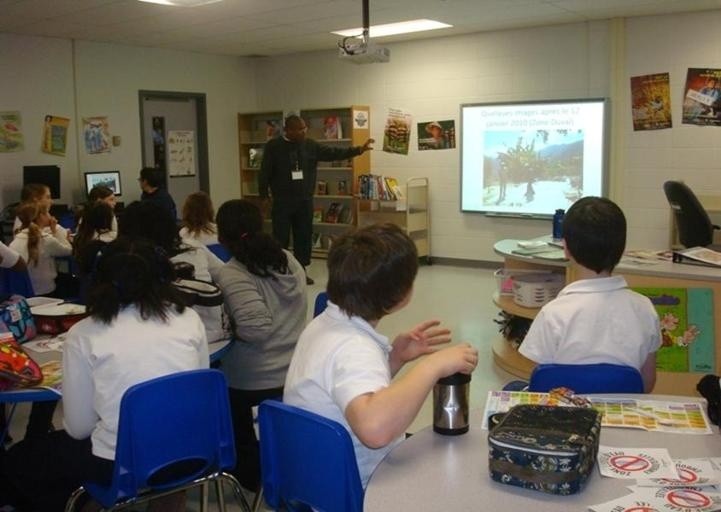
[673,246,721,267]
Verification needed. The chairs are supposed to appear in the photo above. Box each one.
[663,180,721,250]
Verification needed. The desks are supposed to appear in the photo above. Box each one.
[363,393,721,512]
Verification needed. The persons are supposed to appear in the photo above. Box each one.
[424,120,444,148]
[500,195,664,393]
[253,111,375,290]
[281,220,479,488]
[697,73,719,115]
[211,199,309,499]
[1,169,227,307]
[0,234,213,511]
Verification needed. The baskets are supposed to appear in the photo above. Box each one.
[494,270,564,308]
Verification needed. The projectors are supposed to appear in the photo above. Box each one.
[335,37,392,66]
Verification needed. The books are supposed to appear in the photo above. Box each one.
[357,173,403,204]
[248,114,353,248]
[510,237,569,261]
[621,244,721,268]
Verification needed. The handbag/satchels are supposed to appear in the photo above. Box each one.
[489,404,601,495]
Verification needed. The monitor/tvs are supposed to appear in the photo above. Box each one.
[85,171,121,196]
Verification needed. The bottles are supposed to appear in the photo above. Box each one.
[431,370,473,436]
[553,209,567,240]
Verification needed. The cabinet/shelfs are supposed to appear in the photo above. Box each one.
[238,105,369,259]
